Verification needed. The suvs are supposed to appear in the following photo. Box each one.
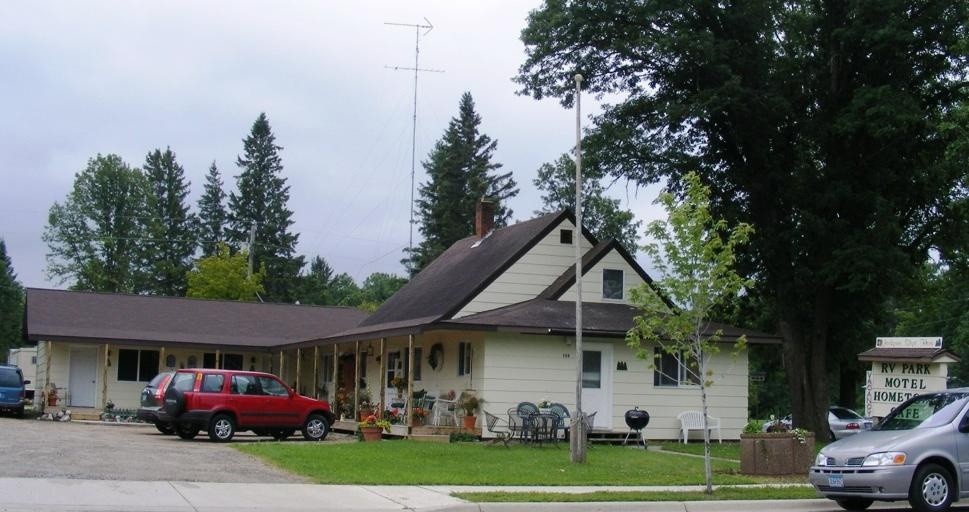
[0,362,31,419]
[137,368,336,444]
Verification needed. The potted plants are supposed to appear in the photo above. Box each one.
[456,392,486,429]
[740,421,815,475]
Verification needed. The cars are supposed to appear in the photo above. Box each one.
[808,386,969,512]
[761,405,874,442]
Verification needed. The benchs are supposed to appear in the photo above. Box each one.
[677,410,722,443]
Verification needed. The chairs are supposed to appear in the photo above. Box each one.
[386,389,464,425]
[483,402,597,450]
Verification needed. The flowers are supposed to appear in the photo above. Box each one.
[358,400,390,441]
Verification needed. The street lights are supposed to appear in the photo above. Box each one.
[569,73,587,464]
